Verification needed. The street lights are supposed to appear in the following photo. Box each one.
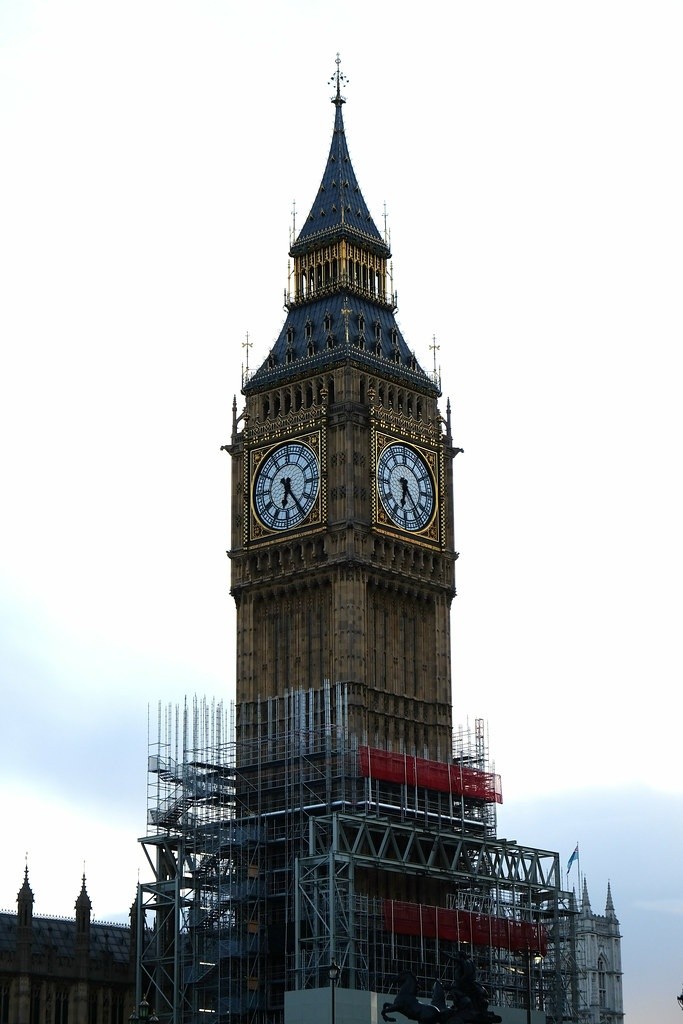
[511,938,542,1024]
[327,957,340,1024]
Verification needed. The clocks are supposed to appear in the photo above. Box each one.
[253,442,317,534]
[379,447,435,533]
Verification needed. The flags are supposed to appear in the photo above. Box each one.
[566,845,579,875]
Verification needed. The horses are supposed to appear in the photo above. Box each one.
[381,970,450,1024]
